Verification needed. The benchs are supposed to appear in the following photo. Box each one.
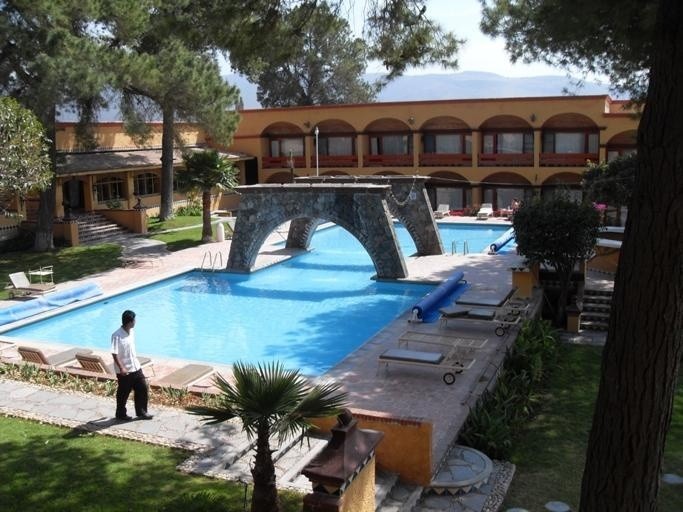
[156,363,216,388]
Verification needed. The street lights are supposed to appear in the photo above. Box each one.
[314,125,320,176]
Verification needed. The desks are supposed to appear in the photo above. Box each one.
[27,266,53,285]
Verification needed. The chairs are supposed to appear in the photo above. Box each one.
[433,203,451,218]
[17,344,154,376]
[438,284,533,331]
[477,202,493,219]
[374,344,476,384]
[1,271,56,298]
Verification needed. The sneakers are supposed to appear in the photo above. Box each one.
[138,413,153,421]
[116,416,132,420]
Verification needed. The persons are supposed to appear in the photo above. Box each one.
[511,198,520,211]
[109,310,155,421]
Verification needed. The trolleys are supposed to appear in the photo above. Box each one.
[28,265,55,286]
